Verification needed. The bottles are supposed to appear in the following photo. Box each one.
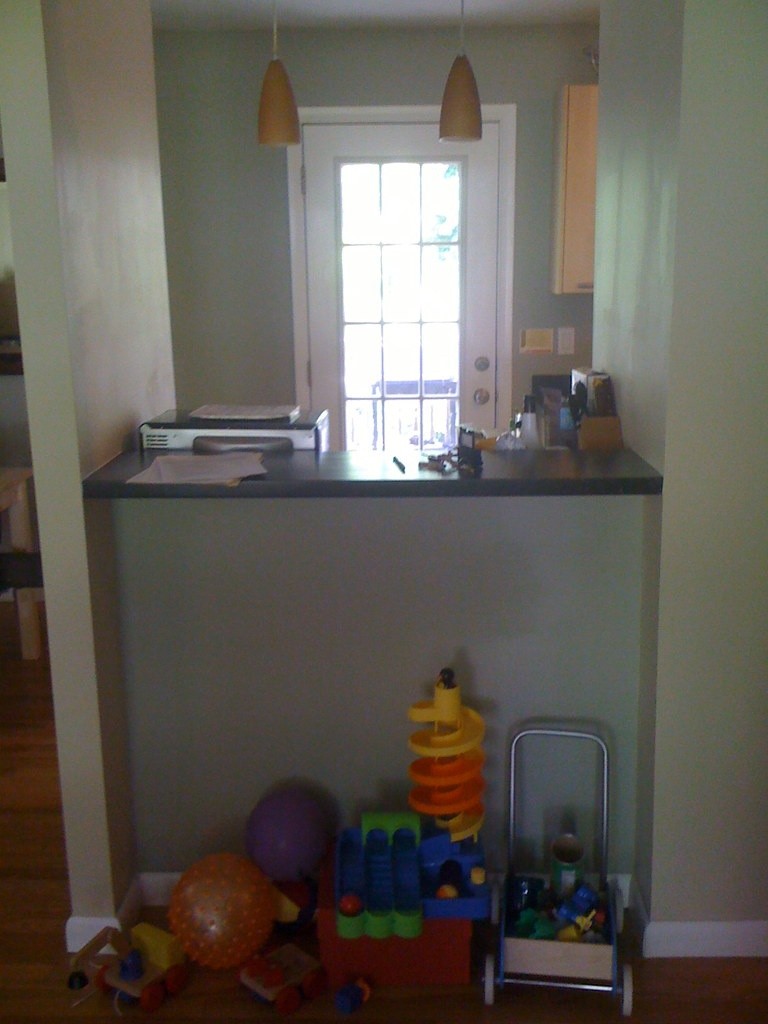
[519,394,541,450]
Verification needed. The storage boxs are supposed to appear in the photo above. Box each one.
[576,417,623,451]
[312,846,469,990]
[572,368,610,415]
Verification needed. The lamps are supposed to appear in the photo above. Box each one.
[258,0,300,147]
[439,0,482,143]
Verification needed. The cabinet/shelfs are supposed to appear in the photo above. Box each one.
[548,83,598,295]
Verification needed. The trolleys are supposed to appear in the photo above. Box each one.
[478,729,634,1015]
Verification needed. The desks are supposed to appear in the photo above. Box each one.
[0,466,42,659]
